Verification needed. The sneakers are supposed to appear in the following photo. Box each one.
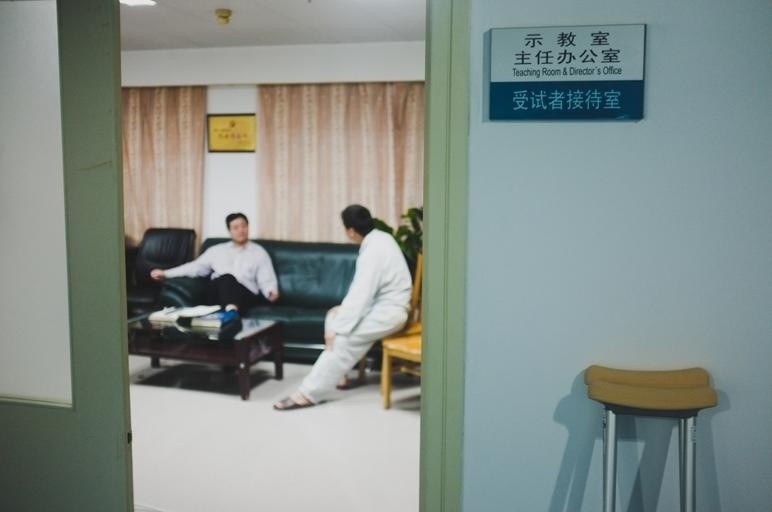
[217,315,244,344]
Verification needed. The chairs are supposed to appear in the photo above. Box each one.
[379,254,424,409]
[124,226,196,317]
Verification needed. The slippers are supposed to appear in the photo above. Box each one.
[335,383,351,390]
[273,394,328,413]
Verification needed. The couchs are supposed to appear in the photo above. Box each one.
[188,238,384,374]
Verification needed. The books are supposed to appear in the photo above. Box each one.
[190,310,237,328]
[147,305,222,324]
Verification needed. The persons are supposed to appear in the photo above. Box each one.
[273,203,414,411]
[149,212,281,375]
